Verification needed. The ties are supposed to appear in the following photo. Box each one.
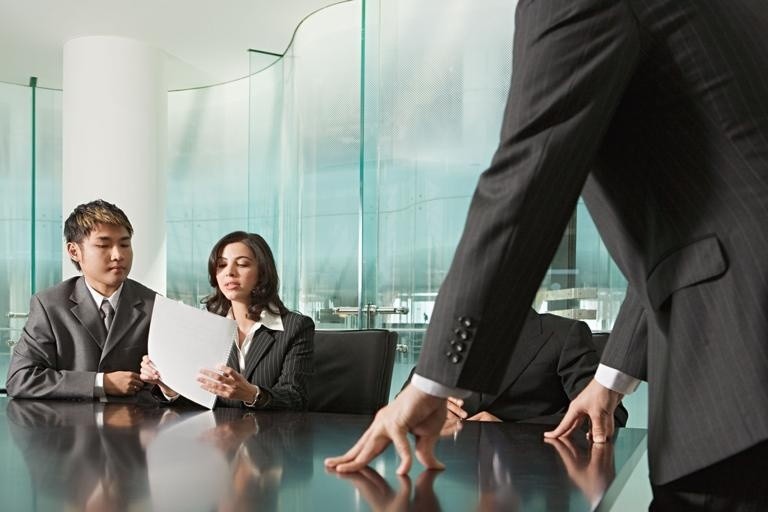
[101,301,113,333]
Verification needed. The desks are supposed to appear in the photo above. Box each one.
[0,395,646,512]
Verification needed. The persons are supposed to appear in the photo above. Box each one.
[323,0,768,512]
[394,306,629,429]
[139,231,316,410]
[5,200,163,399]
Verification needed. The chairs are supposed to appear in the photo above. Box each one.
[312,329,398,416]
[593,331,611,357]
